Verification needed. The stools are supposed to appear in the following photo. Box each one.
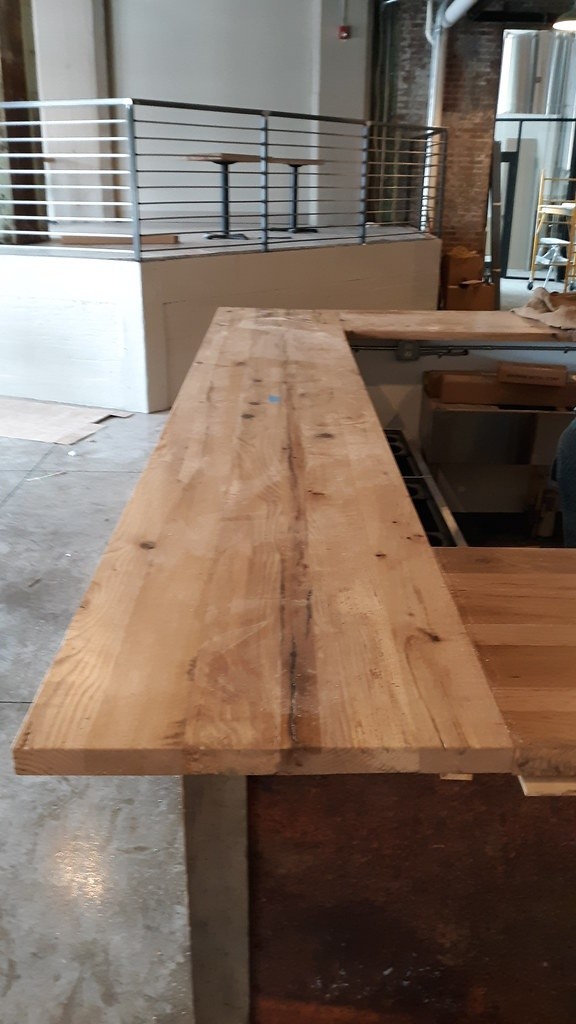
[264,158,324,233]
[183,153,261,239]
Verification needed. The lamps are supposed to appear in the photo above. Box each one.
[552,0,576,31]
